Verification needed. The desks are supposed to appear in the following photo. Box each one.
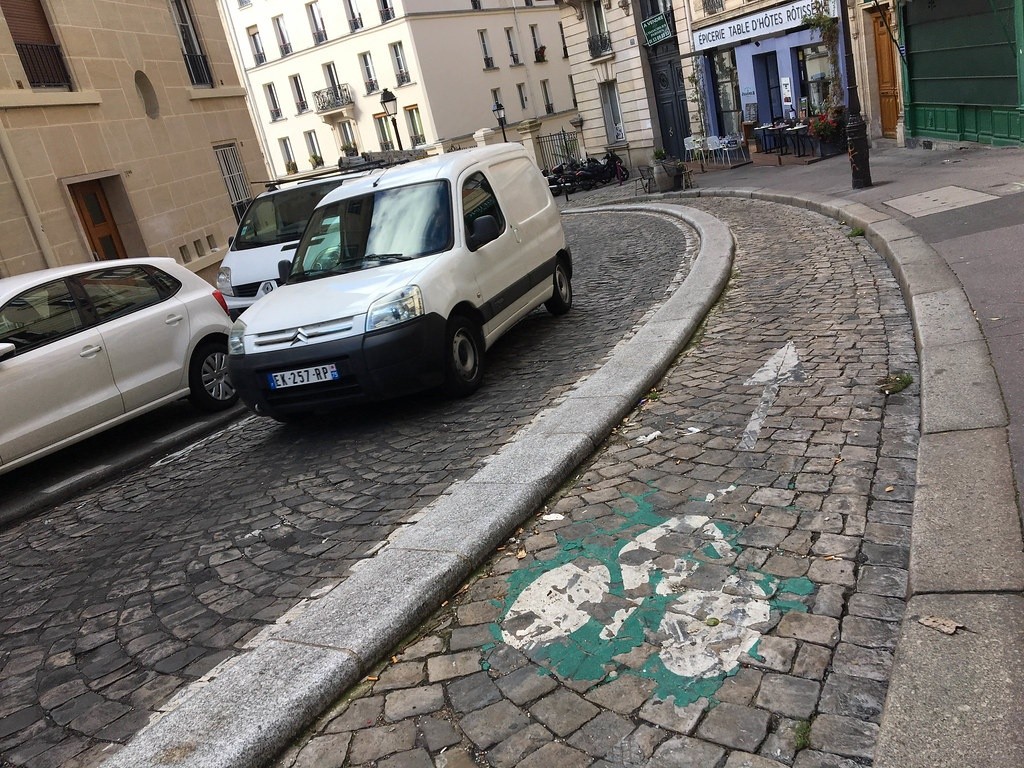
[755,122,814,157]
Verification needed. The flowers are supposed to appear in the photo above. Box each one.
[806,110,838,143]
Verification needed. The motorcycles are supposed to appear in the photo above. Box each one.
[541,148,628,196]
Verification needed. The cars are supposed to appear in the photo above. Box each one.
[0,256,240,475]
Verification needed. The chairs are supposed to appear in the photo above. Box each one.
[637,163,656,194]
[684,131,748,167]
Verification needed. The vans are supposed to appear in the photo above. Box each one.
[216,149,428,322]
[226,142,573,426]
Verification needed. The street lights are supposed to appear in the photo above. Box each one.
[491,100,508,143]
[379,88,403,150]
